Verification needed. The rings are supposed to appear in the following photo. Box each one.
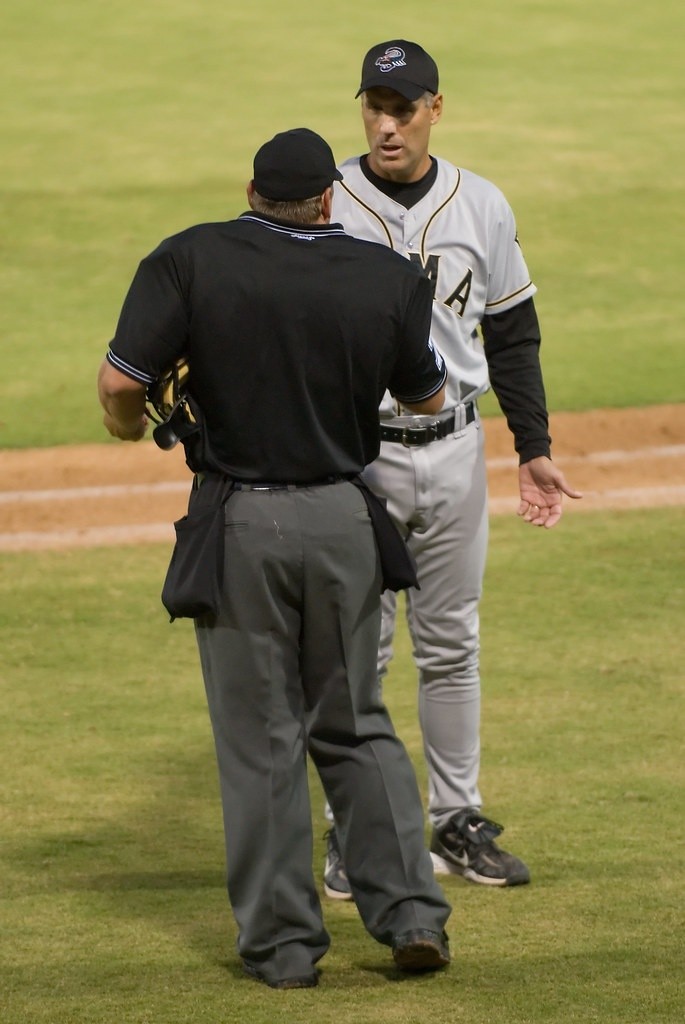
[531,504,538,509]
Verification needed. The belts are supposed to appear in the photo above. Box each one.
[231,474,343,491]
[380,403,475,447]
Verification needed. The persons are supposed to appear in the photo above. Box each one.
[321,41,581,899]
[96,129,453,988]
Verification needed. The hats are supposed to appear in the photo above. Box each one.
[355,40,438,101]
[254,128,344,202]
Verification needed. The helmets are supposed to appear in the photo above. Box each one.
[145,352,196,449]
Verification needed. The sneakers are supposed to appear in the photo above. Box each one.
[243,960,318,988]
[392,929,450,971]
[323,825,353,899]
[429,808,531,886]
